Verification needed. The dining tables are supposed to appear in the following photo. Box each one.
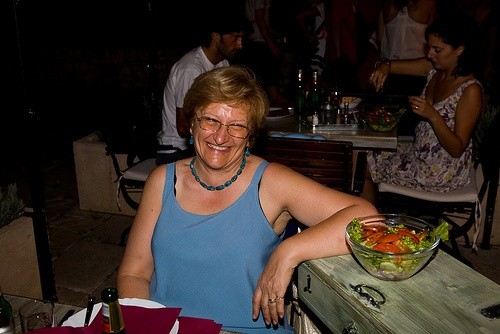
[267,117,398,199]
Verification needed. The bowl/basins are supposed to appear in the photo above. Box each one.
[346,213,440,282]
[340,96,362,109]
[363,107,404,134]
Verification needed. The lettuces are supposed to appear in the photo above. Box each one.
[349,218,449,276]
[369,108,406,131]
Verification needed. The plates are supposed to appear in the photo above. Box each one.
[264,107,294,121]
[61,298,179,334]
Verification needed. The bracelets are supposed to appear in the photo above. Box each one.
[387,60,392,74]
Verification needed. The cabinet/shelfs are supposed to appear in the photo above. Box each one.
[283,242,500,334]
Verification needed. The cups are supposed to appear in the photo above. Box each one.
[19,300,56,331]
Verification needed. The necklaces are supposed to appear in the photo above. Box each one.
[189,146,250,191]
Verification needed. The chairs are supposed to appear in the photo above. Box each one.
[100,128,158,211]
[375,93,497,250]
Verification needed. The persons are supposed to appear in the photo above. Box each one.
[113,64,383,334]
[154,0,500,205]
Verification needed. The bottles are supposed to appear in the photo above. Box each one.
[296,69,350,128]
[0,285,15,334]
[100,288,126,334]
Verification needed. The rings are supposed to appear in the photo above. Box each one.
[275,296,284,300]
[415,106,418,109]
[371,74,375,77]
[268,298,276,303]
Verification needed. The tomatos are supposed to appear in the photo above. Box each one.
[393,240,412,252]
[365,233,383,246]
[377,233,400,243]
[372,243,401,264]
[397,228,410,239]
[398,229,419,244]
[360,231,383,240]
[364,110,392,124]
[418,230,428,240]
[363,226,387,232]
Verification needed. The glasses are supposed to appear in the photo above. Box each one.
[194,112,252,139]
